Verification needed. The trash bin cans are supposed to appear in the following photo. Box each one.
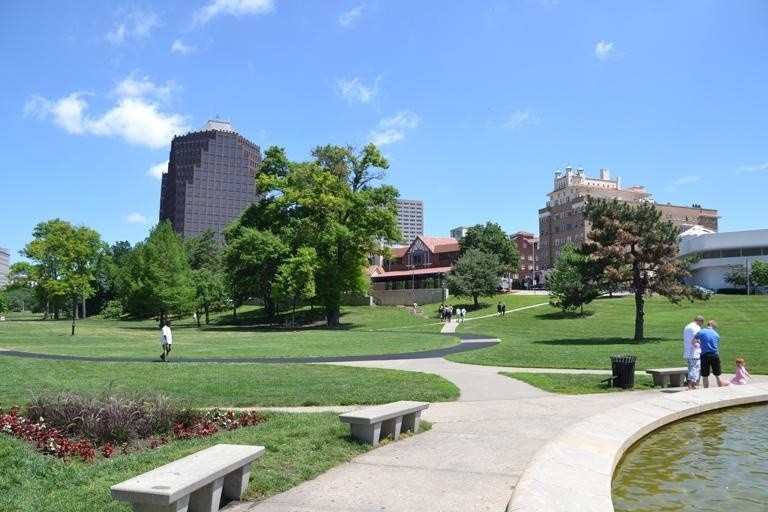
[610,355,637,389]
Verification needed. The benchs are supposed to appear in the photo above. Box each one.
[338,400,428,447]
[108,440,266,512]
[647,366,689,388]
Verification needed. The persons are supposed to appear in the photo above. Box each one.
[439,303,467,324]
[502,300,506,316]
[496,301,501,316]
[691,320,729,388]
[683,315,705,390]
[160,320,172,361]
[730,358,752,385]
[413,301,417,314]
